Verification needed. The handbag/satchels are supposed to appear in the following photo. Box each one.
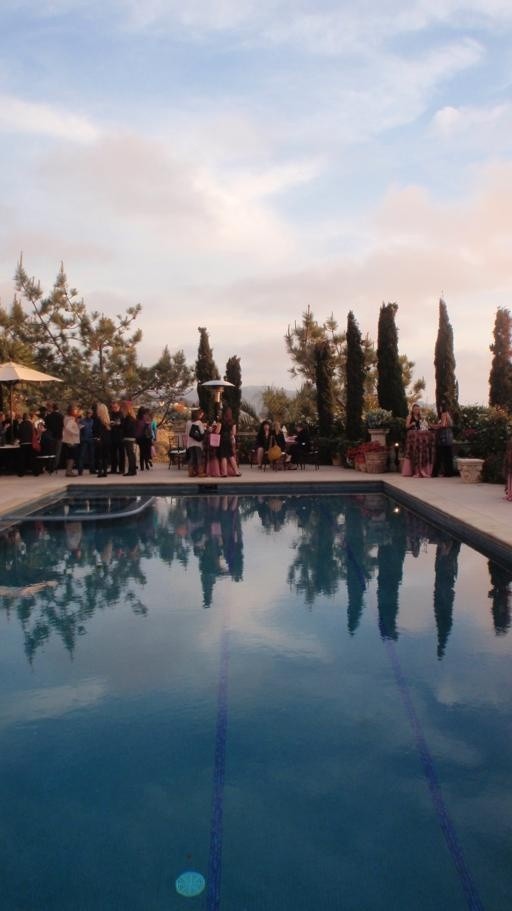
[267,434,282,462]
[188,422,208,442]
[136,422,153,445]
[208,432,221,448]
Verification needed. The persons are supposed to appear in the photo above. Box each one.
[4,500,159,571]
[186,406,241,477]
[406,404,421,430]
[431,403,453,477]
[0,400,156,477]
[256,421,308,468]
[186,496,238,553]
[257,496,311,531]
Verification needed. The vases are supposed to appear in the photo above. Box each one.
[365,451,388,472]
[368,428,391,446]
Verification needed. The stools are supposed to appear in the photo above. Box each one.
[37,455,58,474]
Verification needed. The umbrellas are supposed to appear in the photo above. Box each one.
[0,358,64,444]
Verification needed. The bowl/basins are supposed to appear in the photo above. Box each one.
[431,424,441,429]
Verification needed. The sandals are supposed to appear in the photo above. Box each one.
[288,467,297,470]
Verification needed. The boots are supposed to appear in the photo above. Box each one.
[122,461,138,476]
[65,458,78,477]
[187,464,196,477]
[197,463,208,477]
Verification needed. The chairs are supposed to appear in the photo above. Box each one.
[168,436,186,470]
[299,447,320,471]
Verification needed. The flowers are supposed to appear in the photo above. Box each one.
[367,408,392,428]
[343,442,386,462]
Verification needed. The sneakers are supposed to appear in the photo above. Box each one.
[139,460,153,471]
[88,466,125,478]
[211,472,242,477]
